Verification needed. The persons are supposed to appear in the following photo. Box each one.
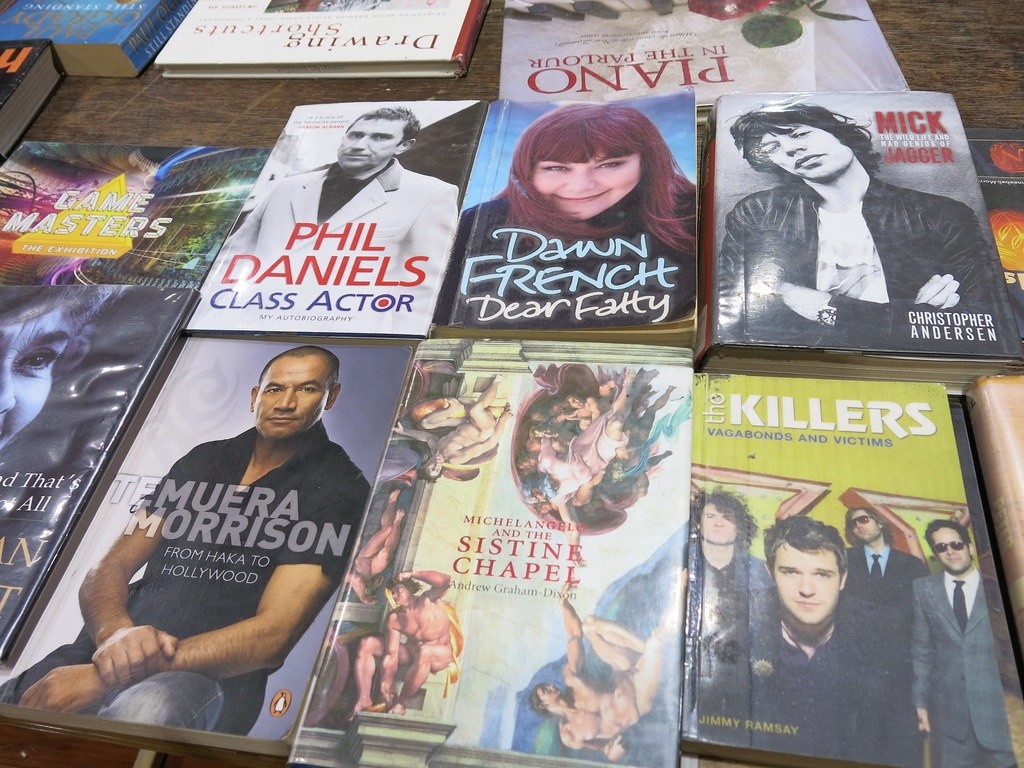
[0,345,371,736]
[836,507,932,705]
[714,514,926,768]
[719,100,1024,356]
[685,484,786,737]
[187,107,459,335]
[912,519,1018,768]
[0,286,135,453]
[432,101,697,329]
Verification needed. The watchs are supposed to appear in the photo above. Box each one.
[816,295,837,329]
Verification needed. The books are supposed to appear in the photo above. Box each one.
[0,0,1024,768]
